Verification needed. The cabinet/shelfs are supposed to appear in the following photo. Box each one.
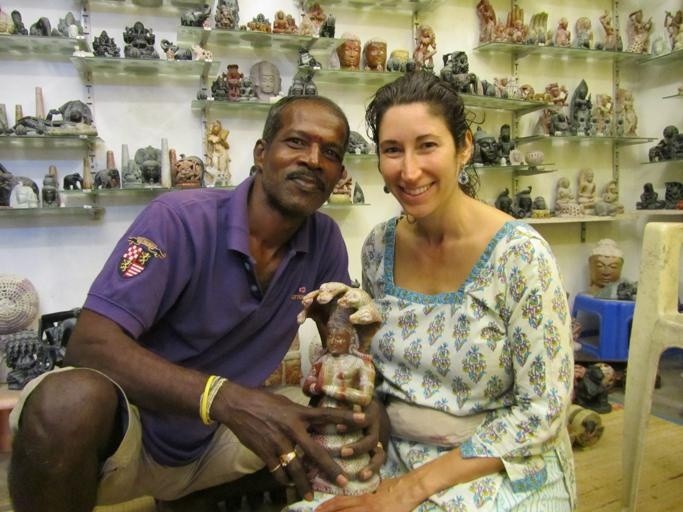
[0,0,683,223]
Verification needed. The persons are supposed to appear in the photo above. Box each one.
[1,0,681,221]
[280,69,578,510]
[298,302,383,500]
[579,238,630,300]
[6,91,388,511]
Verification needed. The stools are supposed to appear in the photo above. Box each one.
[570,292,637,362]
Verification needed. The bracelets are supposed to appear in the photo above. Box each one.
[199,373,229,426]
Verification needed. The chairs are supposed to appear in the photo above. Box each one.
[623,222,683,510]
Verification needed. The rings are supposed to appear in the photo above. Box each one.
[279,452,297,466]
[268,464,284,473]
